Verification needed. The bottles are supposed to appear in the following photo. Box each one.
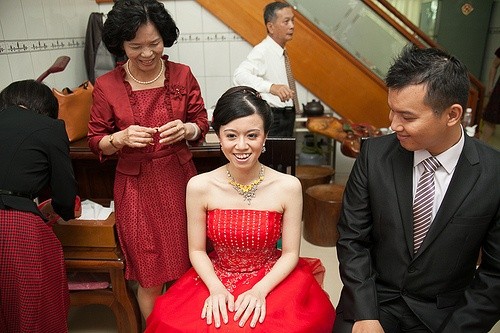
[463,108,472,129]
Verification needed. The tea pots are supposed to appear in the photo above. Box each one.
[301,99,324,116]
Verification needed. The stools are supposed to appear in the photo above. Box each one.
[296,166,332,220]
[303,185,346,247]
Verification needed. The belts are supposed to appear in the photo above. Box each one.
[271,106,295,114]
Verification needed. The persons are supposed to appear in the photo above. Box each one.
[141,86,337,333]
[333,43,500,333]
[479,48,500,135]
[233,3,298,176]
[0,79,81,333]
[87,0,211,322]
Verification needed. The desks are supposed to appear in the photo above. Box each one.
[63,247,138,333]
[69,133,223,199]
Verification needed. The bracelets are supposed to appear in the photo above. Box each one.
[109,135,119,150]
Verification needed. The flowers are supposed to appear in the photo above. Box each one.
[172,82,187,101]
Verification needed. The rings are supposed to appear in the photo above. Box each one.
[206,304,210,308]
[176,127,180,132]
[255,305,262,308]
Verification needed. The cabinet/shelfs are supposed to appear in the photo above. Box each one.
[294,112,336,170]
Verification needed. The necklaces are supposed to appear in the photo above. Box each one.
[225,163,264,204]
[126,58,164,84]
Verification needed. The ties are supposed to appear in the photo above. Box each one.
[283,51,300,114]
[412,156,442,254]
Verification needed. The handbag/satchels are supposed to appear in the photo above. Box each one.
[53,81,96,142]
